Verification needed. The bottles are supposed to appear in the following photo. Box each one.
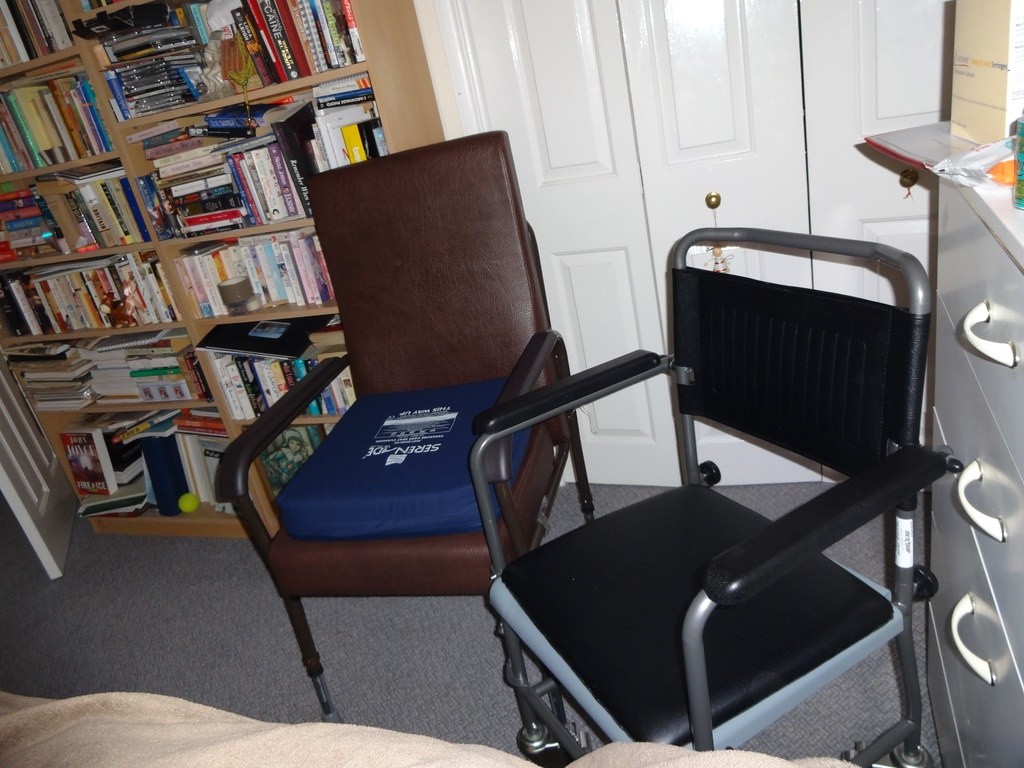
[1013,108,1024,210]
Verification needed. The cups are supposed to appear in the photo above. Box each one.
[217,276,252,304]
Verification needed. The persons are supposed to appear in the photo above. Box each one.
[264,428,310,488]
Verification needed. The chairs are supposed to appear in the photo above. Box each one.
[216,132,595,750]
[467,233,960,768]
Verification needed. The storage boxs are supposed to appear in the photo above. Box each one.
[950,0,1024,148]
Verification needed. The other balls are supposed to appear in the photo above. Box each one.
[178,494,200,514]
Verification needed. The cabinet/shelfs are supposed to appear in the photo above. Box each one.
[0,0,446,541]
[932,143,1024,768]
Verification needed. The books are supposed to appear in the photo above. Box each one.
[80,0,388,171]
[0,0,114,175]
[0,136,388,336]
[59,407,337,517]
[1,314,356,420]
